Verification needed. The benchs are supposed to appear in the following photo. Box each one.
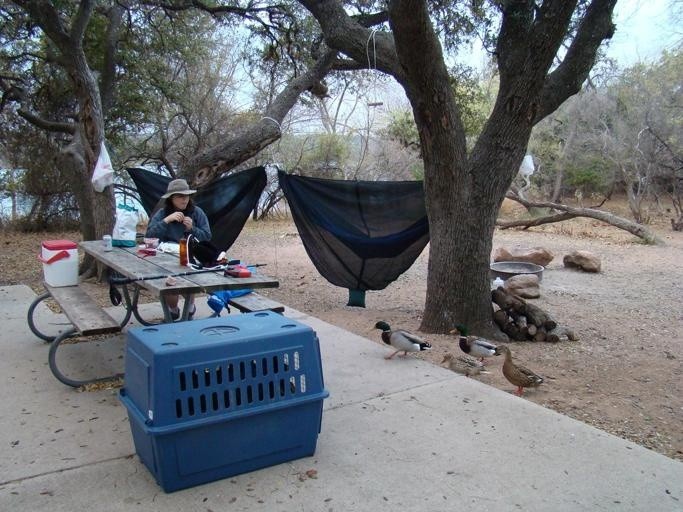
[27,238,286,388]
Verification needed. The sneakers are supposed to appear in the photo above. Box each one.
[187,304,196,314]
[169,307,179,320]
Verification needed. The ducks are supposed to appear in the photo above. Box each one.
[439,352,493,377]
[370,321,432,359]
[493,345,544,395]
[449,323,502,362]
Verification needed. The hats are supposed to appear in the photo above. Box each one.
[159,178,197,199]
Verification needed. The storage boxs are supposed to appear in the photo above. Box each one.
[116,309,330,494]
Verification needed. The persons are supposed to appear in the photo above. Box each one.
[144,178,212,324]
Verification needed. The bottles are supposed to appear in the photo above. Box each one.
[180,237,188,265]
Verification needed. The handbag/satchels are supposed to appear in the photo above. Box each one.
[111,198,139,248]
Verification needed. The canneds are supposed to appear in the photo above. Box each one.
[103,234,112,252]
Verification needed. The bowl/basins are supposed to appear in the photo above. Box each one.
[491,261,544,284]
